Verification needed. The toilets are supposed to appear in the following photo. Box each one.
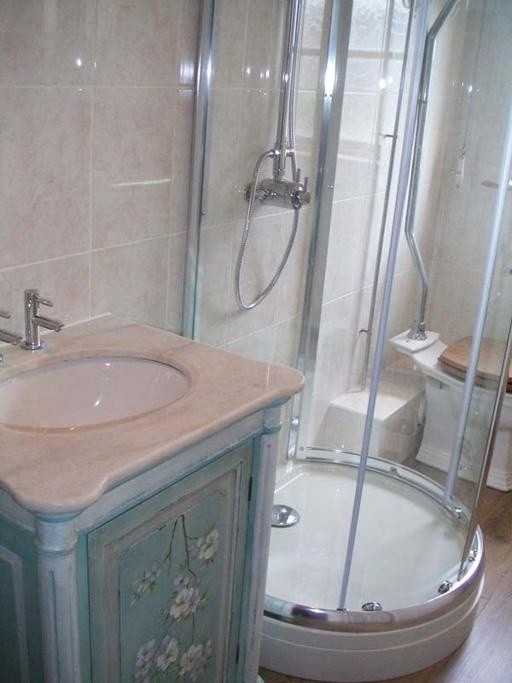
[388,331,512,492]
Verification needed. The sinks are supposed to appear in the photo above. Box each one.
[0,350,192,429]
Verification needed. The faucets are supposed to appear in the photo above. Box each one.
[0,311,24,345]
[23,288,66,349]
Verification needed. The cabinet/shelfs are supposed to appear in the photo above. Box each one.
[1,412,282,682]
